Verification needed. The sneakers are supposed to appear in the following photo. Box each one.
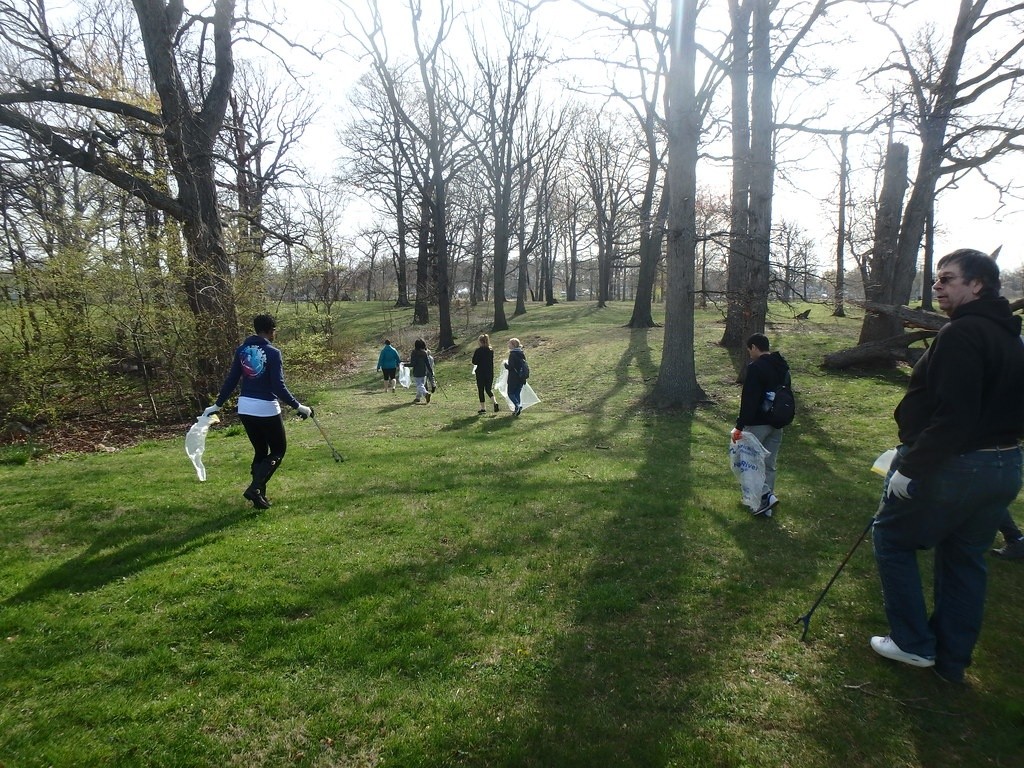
[992,537,1024,561]
[761,509,771,518]
[870,636,936,668]
[751,493,779,515]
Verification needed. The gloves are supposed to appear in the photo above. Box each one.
[887,469,921,506]
[731,428,742,444]
[296,402,314,419]
[203,403,221,417]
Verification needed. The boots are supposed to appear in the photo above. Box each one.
[243,454,281,508]
[251,459,271,509]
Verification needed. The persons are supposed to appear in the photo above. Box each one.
[472,334,500,413]
[990,509,1024,559]
[424,347,436,394]
[869,249,1024,686]
[732,332,792,516]
[402,339,433,404]
[200,314,311,510]
[376,340,401,393]
[503,337,526,416]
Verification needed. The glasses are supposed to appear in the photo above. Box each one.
[931,275,979,286]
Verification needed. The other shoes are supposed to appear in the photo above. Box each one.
[514,404,522,416]
[494,403,499,412]
[478,409,486,414]
[426,393,430,403]
[432,386,436,393]
[413,398,421,404]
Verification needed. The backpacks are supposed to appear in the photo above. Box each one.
[513,351,529,381]
[756,362,794,428]
